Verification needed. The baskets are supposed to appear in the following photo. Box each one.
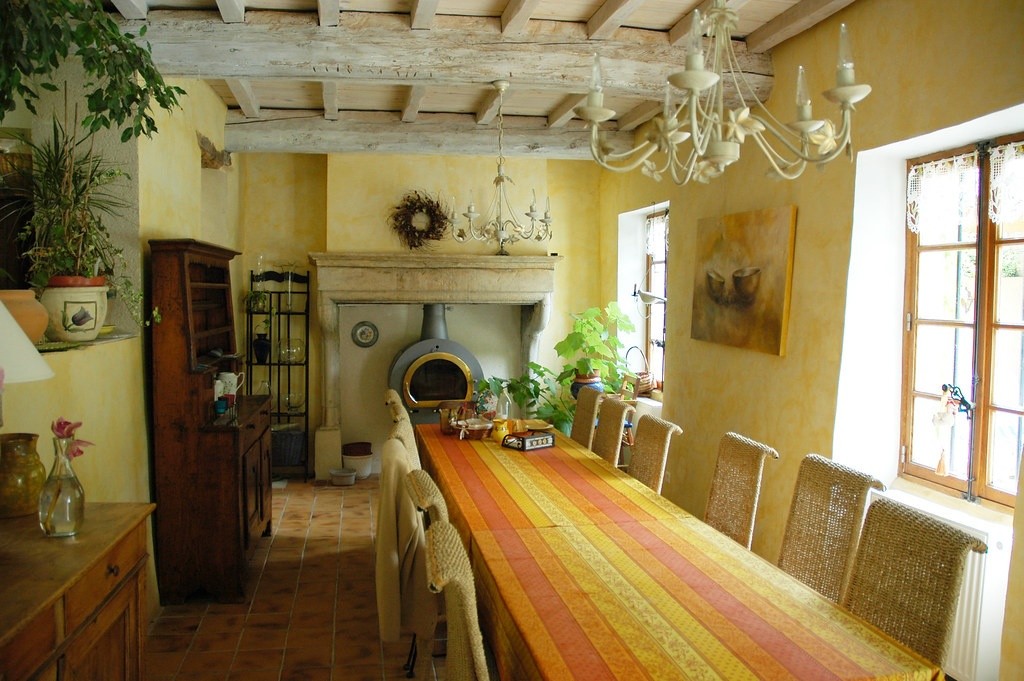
[450,402,494,440]
[627,345,654,393]
[271,430,305,467]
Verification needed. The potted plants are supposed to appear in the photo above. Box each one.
[242,288,271,313]
[554,300,641,401]
[0,81,161,343]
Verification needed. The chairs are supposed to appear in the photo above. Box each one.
[375,389,501,681]
[569,385,988,672]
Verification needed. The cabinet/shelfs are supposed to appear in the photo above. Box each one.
[147,239,273,604]
[247,270,309,484]
[0,504,156,681]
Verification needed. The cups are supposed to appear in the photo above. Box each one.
[218,372,245,405]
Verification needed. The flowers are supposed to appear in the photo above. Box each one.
[43,416,95,534]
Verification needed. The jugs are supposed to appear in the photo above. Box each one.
[491,419,510,442]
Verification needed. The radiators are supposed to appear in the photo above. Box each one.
[870,490,1003,681]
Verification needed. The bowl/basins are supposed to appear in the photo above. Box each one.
[329,467,357,486]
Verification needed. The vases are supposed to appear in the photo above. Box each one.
[38,436,85,537]
[252,334,271,364]
[343,442,374,480]
[0,433,46,520]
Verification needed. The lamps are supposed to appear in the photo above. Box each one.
[571,0,872,186]
[0,301,56,427]
[447,80,554,250]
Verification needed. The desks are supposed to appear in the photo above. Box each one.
[414,419,946,681]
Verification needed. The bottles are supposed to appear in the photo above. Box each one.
[461,402,477,419]
[256,382,277,410]
[497,389,513,438]
[38,436,85,539]
[253,333,270,364]
[0,432,47,520]
